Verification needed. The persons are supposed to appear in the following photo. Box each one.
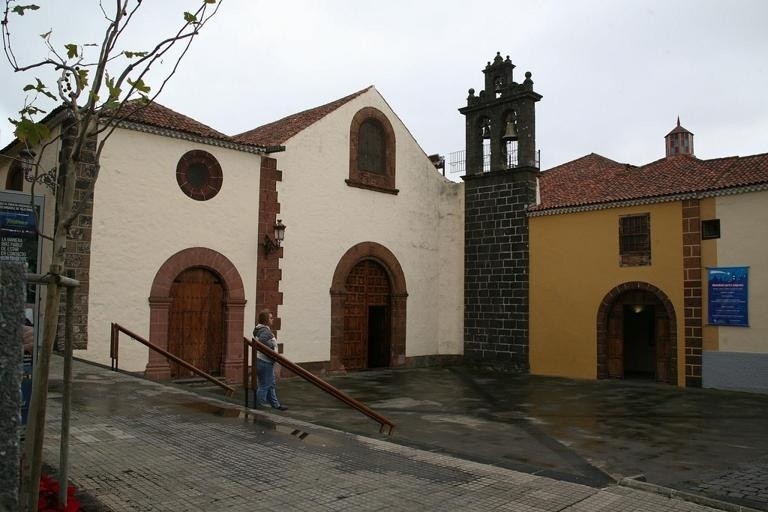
[252,308,289,412]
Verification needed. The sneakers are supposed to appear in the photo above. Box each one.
[277,405,289,410]
